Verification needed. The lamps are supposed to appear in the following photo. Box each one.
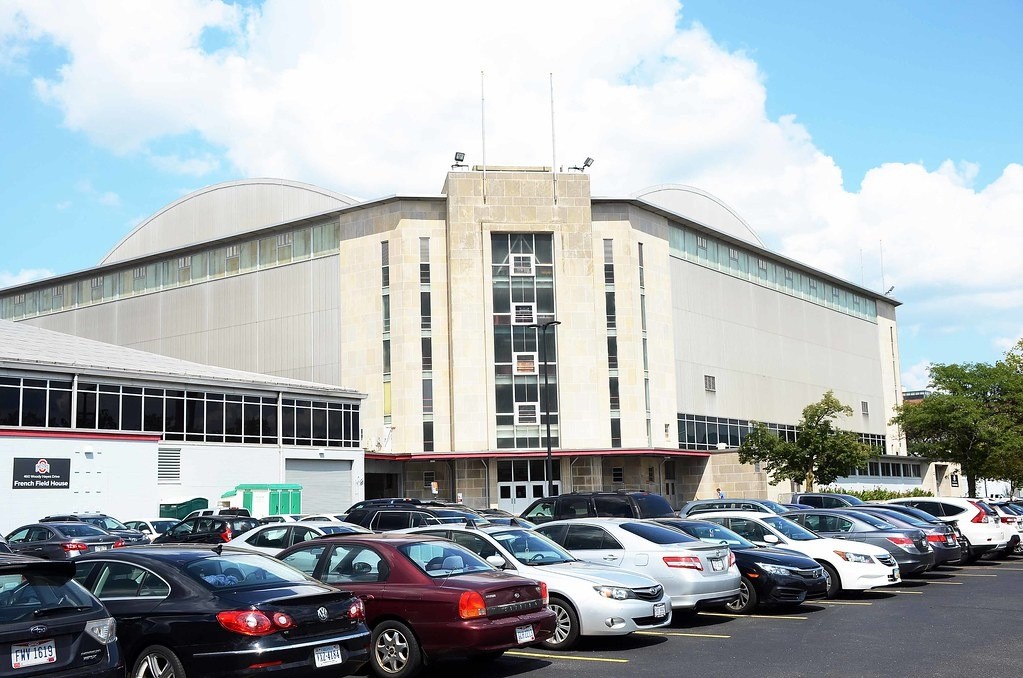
[583,157,594,169]
[455,152,464,165]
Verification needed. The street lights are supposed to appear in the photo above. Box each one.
[527,321,561,496]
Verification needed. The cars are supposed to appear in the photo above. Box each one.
[245,532,557,678]
[381,517,672,651]
[678,499,834,531]
[495,518,741,624]
[5,498,551,577]
[0,535,27,594]
[739,493,1023,578]
[0,543,372,678]
[687,512,902,601]
[642,519,828,615]
[217,521,426,583]
[0,552,126,678]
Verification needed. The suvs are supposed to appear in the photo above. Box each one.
[518,491,677,525]
